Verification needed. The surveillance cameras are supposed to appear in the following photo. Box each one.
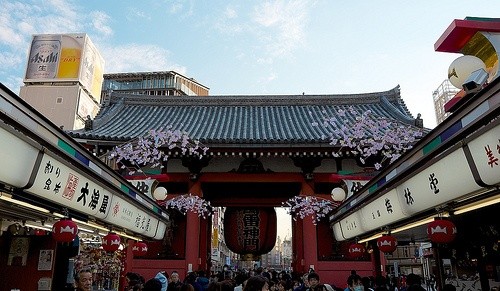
[463,69,489,94]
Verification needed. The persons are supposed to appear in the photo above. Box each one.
[124,263,459,291]
[74,269,95,291]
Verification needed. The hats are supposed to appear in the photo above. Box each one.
[307,272,319,283]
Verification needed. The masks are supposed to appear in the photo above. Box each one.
[351,285,364,291]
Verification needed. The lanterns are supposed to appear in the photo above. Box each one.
[224,206,277,262]
[52,220,79,242]
[131,243,148,255]
[427,217,457,243]
[153,186,168,201]
[377,234,397,253]
[349,244,366,257]
[448,55,487,89]
[331,187,345,202]
[102,233,120,251]
